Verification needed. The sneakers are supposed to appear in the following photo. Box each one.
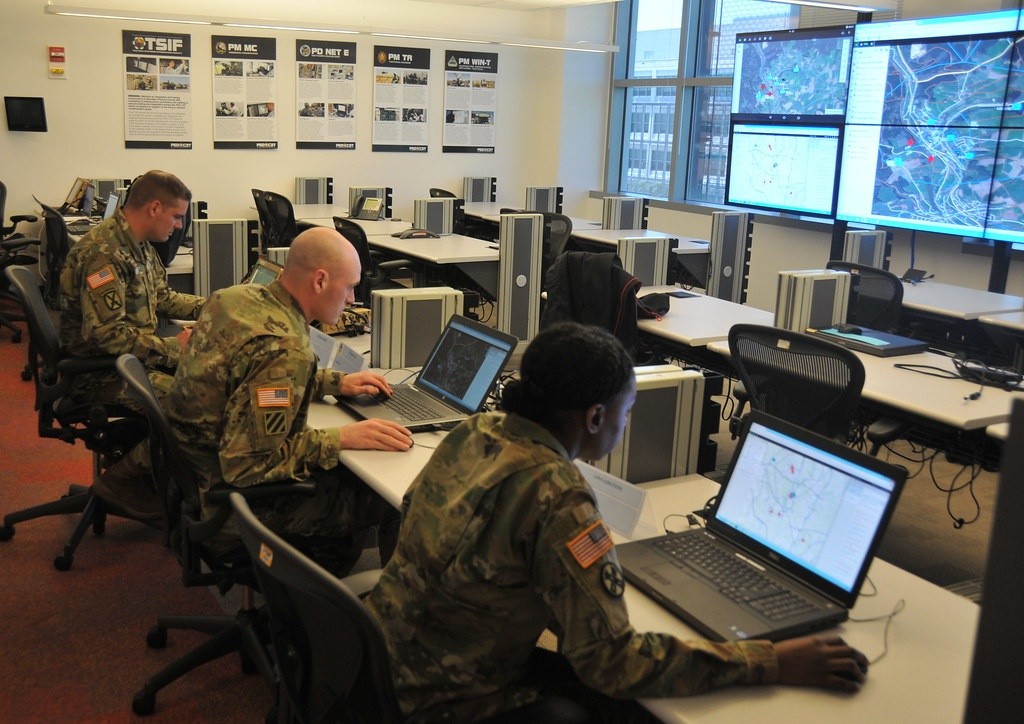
[91,465,163,520]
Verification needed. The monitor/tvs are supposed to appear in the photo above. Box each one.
[4,96,47,133]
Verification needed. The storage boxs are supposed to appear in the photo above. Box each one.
[702,370,724,473]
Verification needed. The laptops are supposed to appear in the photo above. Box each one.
[804,323,930,358]
[67,191,122,236]
[333,313,520,432]
[63,184,95,217]
[614,407,909,644]
[246,256,286,287]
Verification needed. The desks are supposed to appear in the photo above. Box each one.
[300,214,413,236]
[60,213,194,291]
[570,225,711,256]
[461,201,603,231]
[901,276,1024,322]
[977,310,1023,348]
[306,330,981,724]
[632,284,773,346]
[251,203,350,224]
[708,329,1024,430]
[367,231,500,264]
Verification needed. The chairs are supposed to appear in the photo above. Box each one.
[0,262,169,573]
[113,351,281,716]
[225,492,410,724]
[825,261,903,334]
[0,181,42,343]
[239,189,299,284]
[549,252,671,368]
[498,208,573,299]
[331,217,430,309]
[31,193,71,308]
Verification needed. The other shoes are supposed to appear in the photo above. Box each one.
[268,618,291,665]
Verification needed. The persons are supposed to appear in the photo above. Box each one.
[349,324,869,724]
[56,170,208,518]
[164,224,413,565]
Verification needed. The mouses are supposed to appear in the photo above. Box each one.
[838,325,863,335]
[832,659,869,682]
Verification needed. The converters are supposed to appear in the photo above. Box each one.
[961,365,1006,384]
